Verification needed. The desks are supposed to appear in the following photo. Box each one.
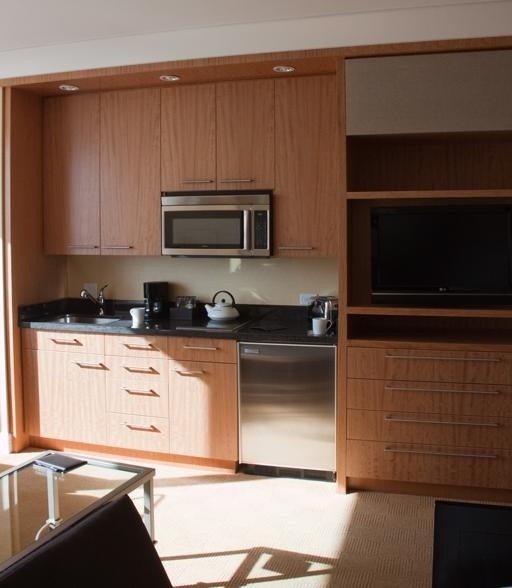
[0,450,156,573]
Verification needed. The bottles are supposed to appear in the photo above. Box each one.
[311,295,338,321]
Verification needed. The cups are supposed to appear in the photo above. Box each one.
[312,319,332,335]
[130,307,146,326]
[176,293,196,309]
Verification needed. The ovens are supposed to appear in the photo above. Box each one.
[237,340,336,483]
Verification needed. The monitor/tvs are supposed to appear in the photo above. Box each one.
[369,204,512,306]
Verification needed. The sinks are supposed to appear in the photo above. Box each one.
[48,312,121,326]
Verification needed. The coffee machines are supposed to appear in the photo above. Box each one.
[144,281,169,321]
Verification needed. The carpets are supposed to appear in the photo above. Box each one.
[0,451,437,588]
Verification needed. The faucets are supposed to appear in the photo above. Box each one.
[79,279,114,318]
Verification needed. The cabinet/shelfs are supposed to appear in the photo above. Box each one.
[273,73,341,257]
[345,346,512,490]
[105,335,169,454]
[167,336,239,465]
[160,77,275,193]
[43,86,162,256]
[20,326,105,446]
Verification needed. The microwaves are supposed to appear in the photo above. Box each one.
[161,193,273,259]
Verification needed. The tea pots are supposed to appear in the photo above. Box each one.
[204,291,239,322]
